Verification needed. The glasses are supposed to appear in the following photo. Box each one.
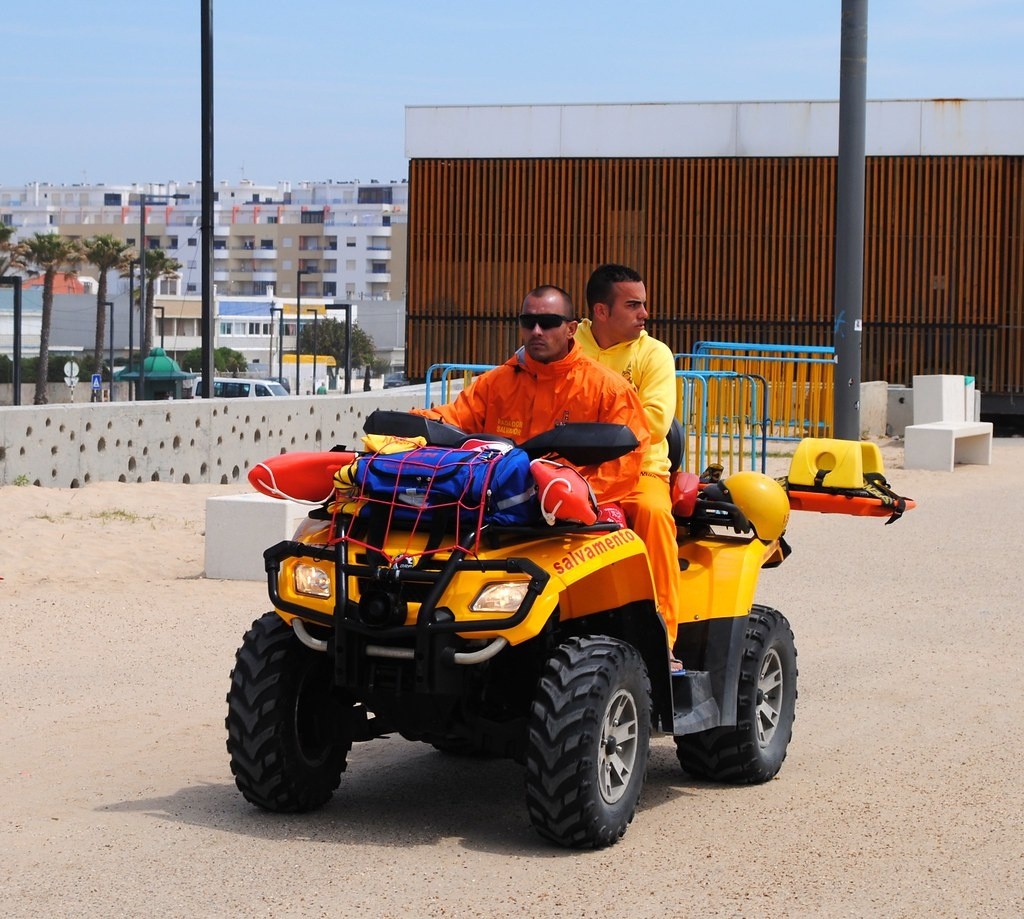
[519,313,573,330]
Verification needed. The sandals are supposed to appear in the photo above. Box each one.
[669,657,687,676]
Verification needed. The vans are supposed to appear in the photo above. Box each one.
[190,377,291,400]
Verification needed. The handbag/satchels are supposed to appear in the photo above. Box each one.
[346,447,540,570]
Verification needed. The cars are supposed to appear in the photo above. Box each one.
[384,369,411,389]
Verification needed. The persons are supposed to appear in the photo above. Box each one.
[409,283,650,530]
[568,260,684,678]
